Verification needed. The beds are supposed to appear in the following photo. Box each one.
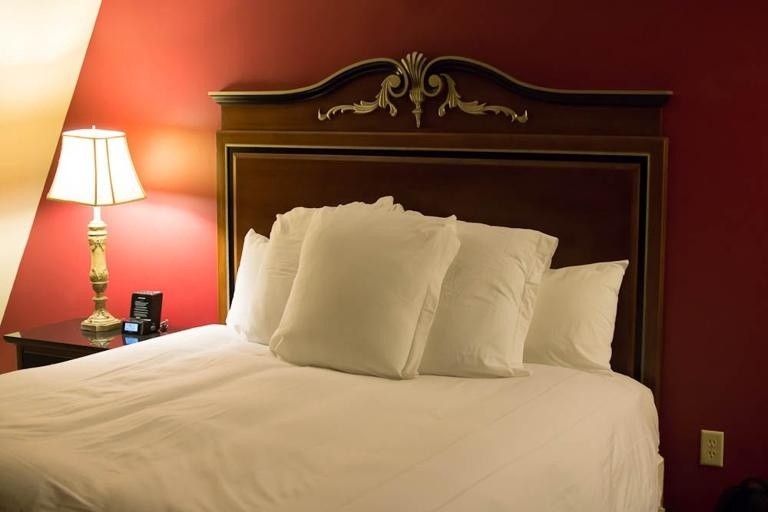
[0,61,682,511]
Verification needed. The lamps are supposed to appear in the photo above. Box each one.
[47,121,148,335]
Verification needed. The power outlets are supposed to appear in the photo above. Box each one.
[698,425,726,471]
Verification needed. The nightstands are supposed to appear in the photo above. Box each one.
[3,312,176,370]
